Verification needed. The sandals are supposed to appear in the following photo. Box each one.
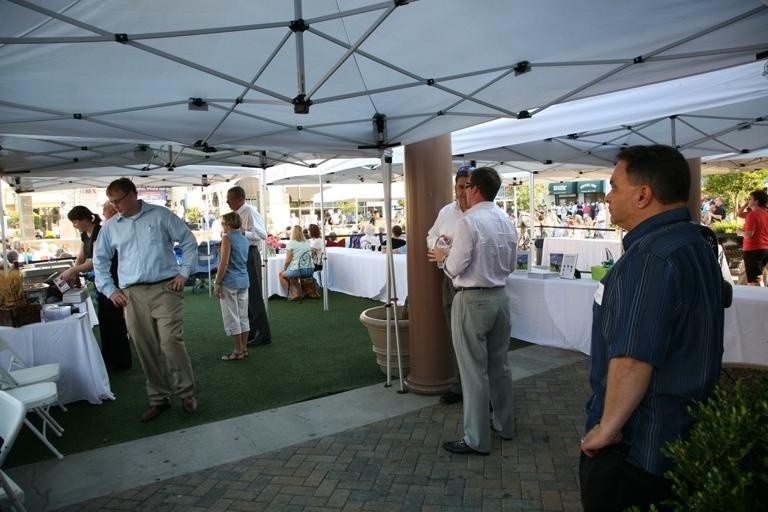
[220,351,249,361]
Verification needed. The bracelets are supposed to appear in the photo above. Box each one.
[442,255,448,263]
[214,280,220,284]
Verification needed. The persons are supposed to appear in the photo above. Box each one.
[578,146,725,512]
[92,178,198,421]
[213,213,250,361]
[280,209,407,300]
[427,167,518,455]
[61,206,132,372]
[102,201,117,221]
[221,187,273,345]
[507,201,623,249]
[0,231,70,275]
[700,188,768,287]
[425,167,494,413]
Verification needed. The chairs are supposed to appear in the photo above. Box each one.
[0,391,27,512]
[0,367,63,460]
[190,267,217,294]
[0,335,68,432]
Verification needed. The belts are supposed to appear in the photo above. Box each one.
[457,287,488,291]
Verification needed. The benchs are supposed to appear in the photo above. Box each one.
[539,236,624,273]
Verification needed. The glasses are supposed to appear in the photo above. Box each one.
[458,166,476,172]
[109,193,128,205]
[465,183,471,188]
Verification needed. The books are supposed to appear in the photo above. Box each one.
[527,270,560,280]
[62,287,88,303]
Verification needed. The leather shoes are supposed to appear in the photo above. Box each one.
[491,418,512,440]
[440,439,490,456]
[440,392,463,404]
[183,396,197,413]
[247,335,272,347]
[143,399,171,420]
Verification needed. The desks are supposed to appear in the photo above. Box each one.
[508,271,601,357]
[262,239,304,300]
[321,245,409,308]
[719,284,768,365]
[0,258,116,412]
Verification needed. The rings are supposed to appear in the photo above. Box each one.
[580,436,584,443]
